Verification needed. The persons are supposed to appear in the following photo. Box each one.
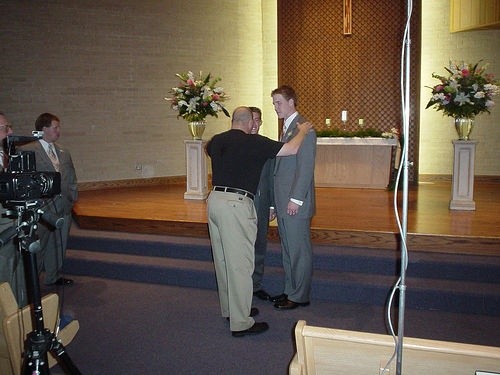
[0,113,79,306]
[271,86,317,310]
[206,106,313,336]
[247,106,276,300]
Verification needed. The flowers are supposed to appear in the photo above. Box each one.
[165,72,230,118]
[422,57,500,119]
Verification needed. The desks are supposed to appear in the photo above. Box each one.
[314,136,398,190]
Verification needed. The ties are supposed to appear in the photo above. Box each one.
[48,144,57,163]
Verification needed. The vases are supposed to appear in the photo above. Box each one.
[188,120,206,140]
[453,116,474,140]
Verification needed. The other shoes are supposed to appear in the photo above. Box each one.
[232,322,268,337]
[272,293,290,302]
[253,290,270,300]
[227,307,258,321]
[45,277,73,287]
[274,299,309,309]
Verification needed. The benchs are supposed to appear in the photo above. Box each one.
[288,319,500,375]
[0,279,81,375]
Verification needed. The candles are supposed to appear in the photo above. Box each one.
[326,118,331,125]
[359,119,364,125]
[342,111,347,121]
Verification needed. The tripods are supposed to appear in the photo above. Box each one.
[0,201,83,375]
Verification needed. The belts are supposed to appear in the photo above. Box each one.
[212,187,254,200]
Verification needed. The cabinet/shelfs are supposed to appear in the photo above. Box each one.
[450,0,500,34]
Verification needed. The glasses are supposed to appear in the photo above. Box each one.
[0,124,12,132]
